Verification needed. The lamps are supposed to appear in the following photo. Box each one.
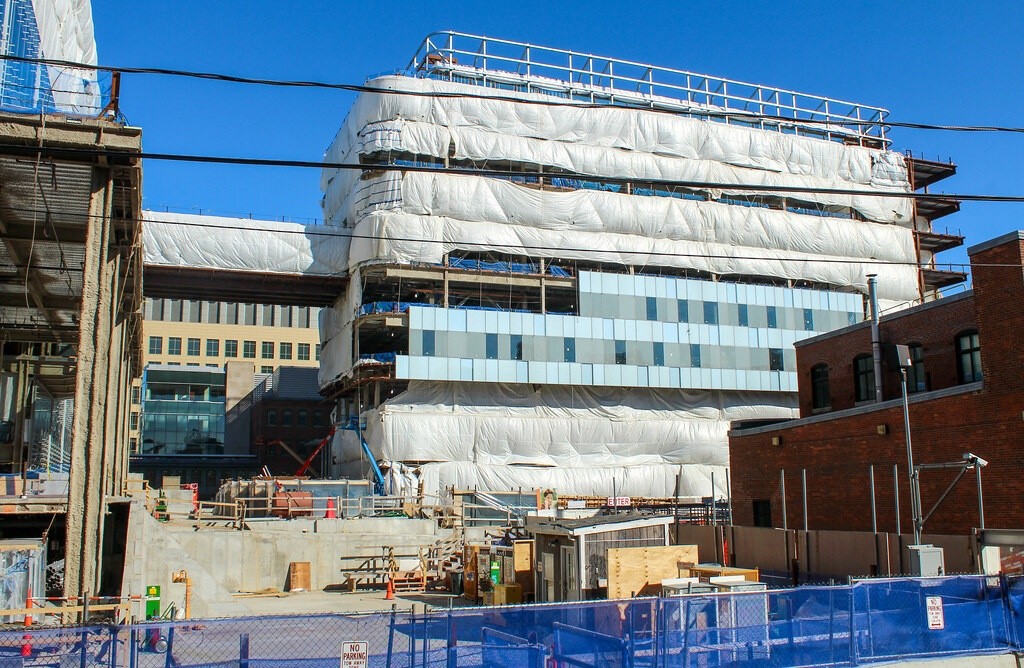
[771,436,781,446]
[876,424,888,435]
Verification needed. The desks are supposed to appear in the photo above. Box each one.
[341,553,418,565]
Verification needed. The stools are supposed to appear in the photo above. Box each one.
[343,567,401,593]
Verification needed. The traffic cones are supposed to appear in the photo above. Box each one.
[384,582,395,600]
[324,496,336,518]
